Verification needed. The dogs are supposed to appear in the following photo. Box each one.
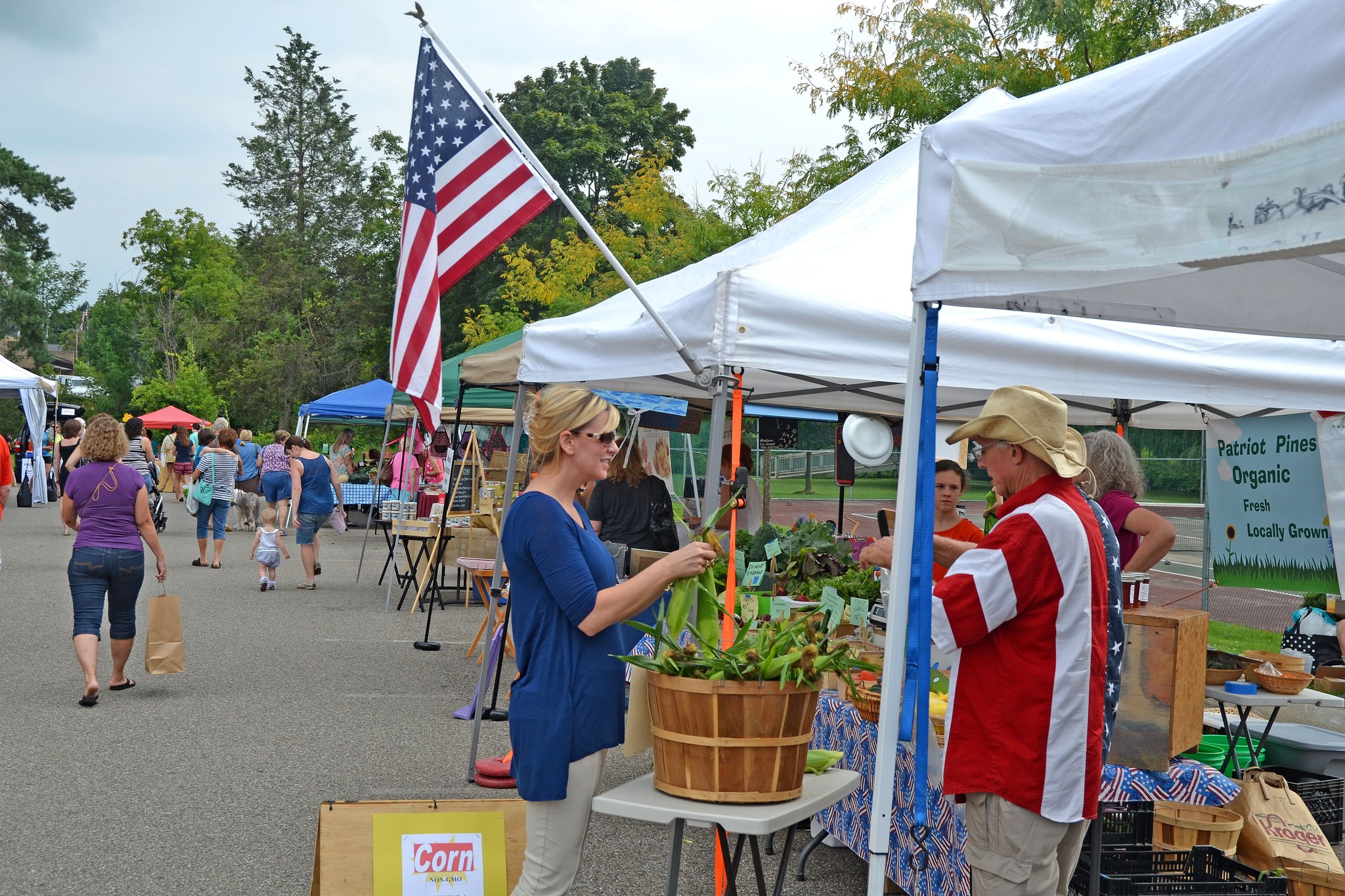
[232,488,260,533]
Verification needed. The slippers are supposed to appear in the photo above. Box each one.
[211,562,221,568]
[192,557,209,567]
[346,522,357,527]
[79,694,99,706]
[110,676,136,690]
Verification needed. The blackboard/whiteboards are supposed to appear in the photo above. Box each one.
[446,460,483,516]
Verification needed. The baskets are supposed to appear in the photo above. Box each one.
[1206,668,1247,685]
[1253,668,1315,695]
[848,678,948,747]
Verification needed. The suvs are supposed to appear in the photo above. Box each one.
[15,401,84,484]
[44,375,106,398]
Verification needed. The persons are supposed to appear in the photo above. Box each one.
[1072,428,1176,573]
[680,444,764,552]
[284,435,345,591]
[930,459,985,671]
[191,429,239,569]
[983,479,1007,538]
[499,383,718,896]
[460,441,491,478]
[379,435,420,544]
[256,429,295,536]
[249,508,290,592]
[414,452,426,484]
[587,437,679,579]
[231,430,263,525]
[574,479,600,511]
[420,441,445,486]
[858,386,1123,896]
[60,415,167,707]
[367,448,384,469]
[0,404,245,536]
[329,428,358,527]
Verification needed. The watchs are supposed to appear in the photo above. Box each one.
[1122,569,1125,573]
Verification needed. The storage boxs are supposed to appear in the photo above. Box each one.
[1313,666,1345,694]
[1067,706,1345,896]
[392,520,438,537]
[472,573,510,605]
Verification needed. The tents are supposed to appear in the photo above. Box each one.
[270,378,395,529]
[463,84,1345,786]
[866,0,1343,894]
[0,352,60,509]
[409,337,533,733]
[353,321,529,612]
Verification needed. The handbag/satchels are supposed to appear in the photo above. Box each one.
[161,452,167,468]
[255,442,263,476]
[1280,607,1342,685]
[648,474,680,553]
[144,579,185,675]
[192,452,215,506]
[1223,766,1345,881]
[379,451,399,487]
[182,476,199,514]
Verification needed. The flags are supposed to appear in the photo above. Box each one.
[387,25,558,440]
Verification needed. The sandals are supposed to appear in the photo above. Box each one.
[279,530,289,536]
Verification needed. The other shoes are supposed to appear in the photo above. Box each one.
[63,530,70,535]
[208,524,232,532]
[158,485,184,503]
[260,578,275,591]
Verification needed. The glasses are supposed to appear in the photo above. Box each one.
[971,440,1003,461]
[569,429,617,445]
[51,426,59,429]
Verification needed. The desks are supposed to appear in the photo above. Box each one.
[373,519,410,589]
[401,524,503,608]
[330,478,393,519]
[591,765,861,896]
[1205,679,1345,781]
[416,487,439,520]
[392,534,455,613]
[462,566,516,664]
[155,461,194,492]
[806,686,1243,896]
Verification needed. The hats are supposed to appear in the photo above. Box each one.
[946,385,1097,499]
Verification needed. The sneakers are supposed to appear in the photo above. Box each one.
[296,581,316,590]
[314,560,321,575]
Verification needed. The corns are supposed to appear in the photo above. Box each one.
[650,527,837,699]
